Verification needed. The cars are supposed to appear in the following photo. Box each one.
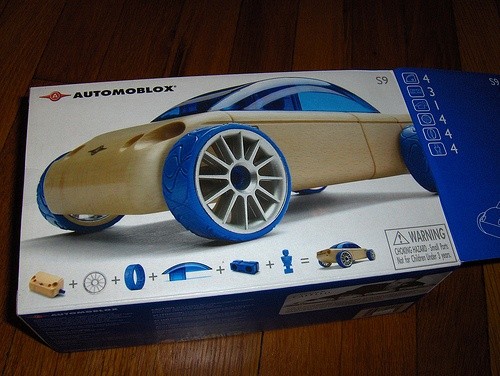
[317,240,377,268]
[36,75,441,244]
